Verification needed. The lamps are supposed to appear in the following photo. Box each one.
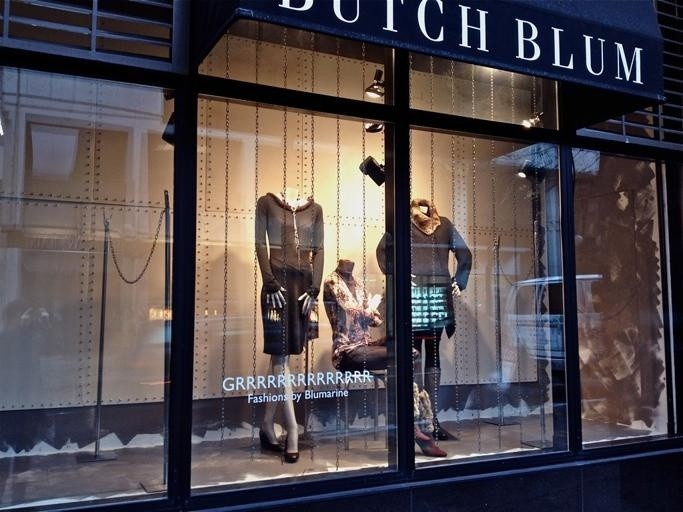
[358,69,386,187]
[517,159,547,185]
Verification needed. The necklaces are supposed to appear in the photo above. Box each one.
[280,193,303,268]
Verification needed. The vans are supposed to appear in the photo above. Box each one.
[499,274,606,369]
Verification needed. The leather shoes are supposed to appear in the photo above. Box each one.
[284,436,299,463]
[433,428,459,441]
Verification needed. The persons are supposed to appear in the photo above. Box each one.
[254,186,325,462]
[376,198,472,441]
[323,258,458,457]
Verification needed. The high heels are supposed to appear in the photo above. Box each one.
[259,426,286,452]
[415,431,448,457]
